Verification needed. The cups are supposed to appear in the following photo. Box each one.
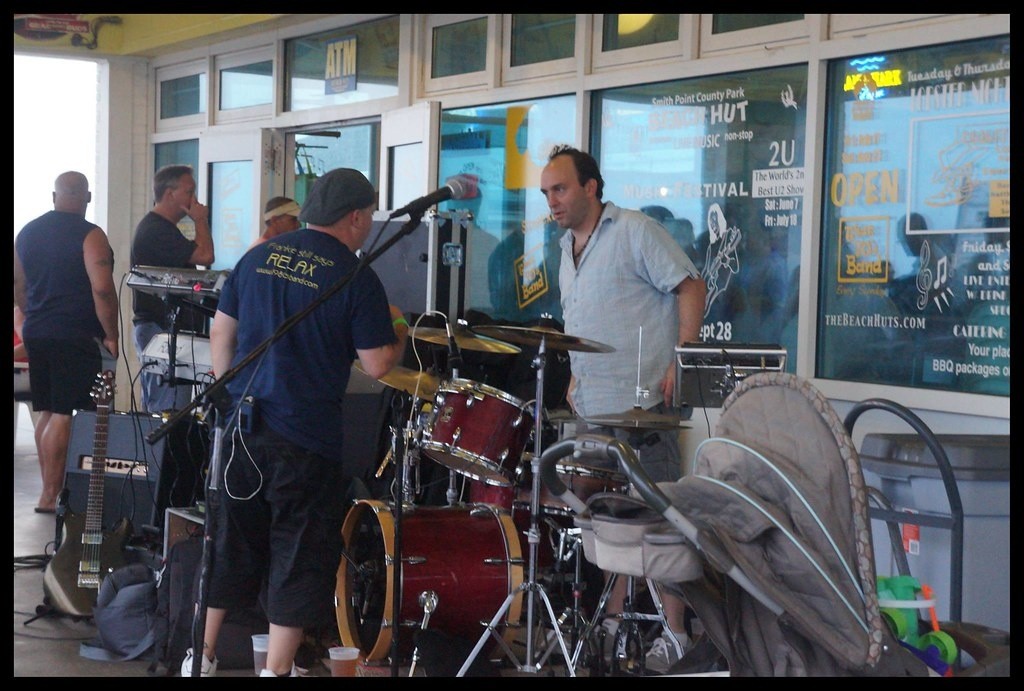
[328,647,360,677]
[250,633,270,676]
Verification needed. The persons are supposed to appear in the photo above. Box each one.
[248,197,301,254]
[13,171,119,515]
[181,168,410,677]
[541,145,709,671]
[131,165,215,412]
[446,181,1010,399]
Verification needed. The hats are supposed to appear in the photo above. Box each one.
[299,169,376,225]
[264,200,302,221]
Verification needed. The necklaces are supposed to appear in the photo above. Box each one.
[572,208,603,258]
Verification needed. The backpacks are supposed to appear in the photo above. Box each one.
[148,528,269,677]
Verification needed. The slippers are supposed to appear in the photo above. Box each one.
[35,507,55,513]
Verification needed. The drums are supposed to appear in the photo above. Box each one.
[470,449,589,584]
[420,377,537,489]
[334,496,525,664]
[515,459,630,519]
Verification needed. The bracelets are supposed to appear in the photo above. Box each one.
[392,318,410,330]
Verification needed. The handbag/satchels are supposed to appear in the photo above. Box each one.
[79,565,157,661]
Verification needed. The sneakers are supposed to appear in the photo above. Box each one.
[593,619,643,658]
[182,647,218,677]
[259,660,318,677]
[626,634,693,675]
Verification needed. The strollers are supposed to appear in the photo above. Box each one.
[538,370,1008,680]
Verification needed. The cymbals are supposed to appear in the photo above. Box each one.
[353,360,443,403]
[471,322,618,355]
[408,325,524,355]
[583,407,694,432]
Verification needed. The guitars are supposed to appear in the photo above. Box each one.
[43,369,134,621]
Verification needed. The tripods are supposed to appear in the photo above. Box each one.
[457,337,685,678]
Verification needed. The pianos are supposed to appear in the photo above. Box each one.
[125,262,230,387]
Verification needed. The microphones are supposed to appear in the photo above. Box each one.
[445,316,462,369]
[389,179,467,219]
